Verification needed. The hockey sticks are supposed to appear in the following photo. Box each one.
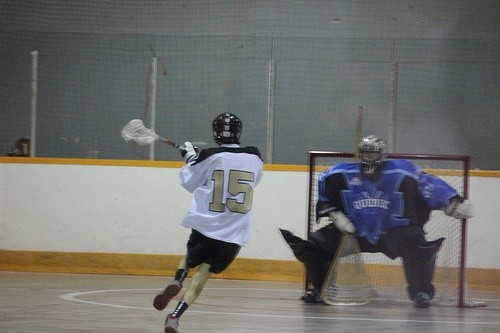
[121,119,200,156]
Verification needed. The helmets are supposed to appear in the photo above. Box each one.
[213,113,242,144]
[359,135,388,174]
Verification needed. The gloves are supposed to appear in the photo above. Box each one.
[332,211,355,233]
[181,142,196,162]
[445,199,474,219]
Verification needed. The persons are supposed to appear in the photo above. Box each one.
[152,111,266,333]
[279,133,474,310]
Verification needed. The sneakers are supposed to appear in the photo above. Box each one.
[153,281,182,310]
[415,292,431,308]
[164,314,179,333]
[302,287,336,303]
